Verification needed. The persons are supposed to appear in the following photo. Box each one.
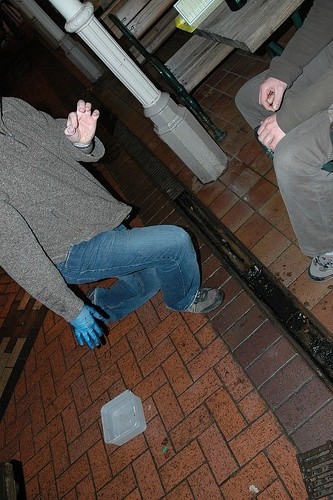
[235,0,333,282]
[1,93,226,350]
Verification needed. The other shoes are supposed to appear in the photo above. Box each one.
[165,287,224,313]
[306,253,333,282]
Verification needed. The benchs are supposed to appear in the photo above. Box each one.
[108,0,235,143]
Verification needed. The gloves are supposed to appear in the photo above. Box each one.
[69,305,107,349]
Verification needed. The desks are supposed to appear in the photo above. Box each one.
[175,0,305,54]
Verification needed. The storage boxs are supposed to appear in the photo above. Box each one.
[101,389,147,446]
